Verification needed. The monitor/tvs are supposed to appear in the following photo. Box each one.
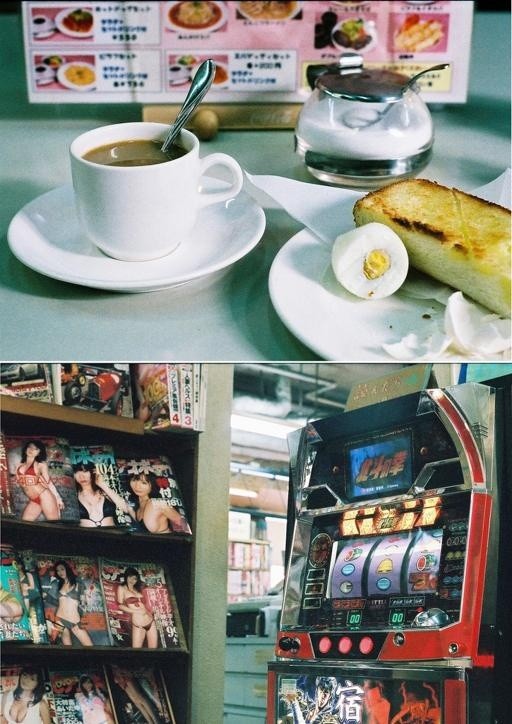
[346,426,412,500]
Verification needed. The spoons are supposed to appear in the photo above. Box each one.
[336,63,450,129]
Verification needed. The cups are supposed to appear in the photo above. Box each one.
[67,121,248,263]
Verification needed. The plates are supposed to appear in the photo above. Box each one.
[5,169,268,301]
[266,196,511,362]
[32,0,450,95]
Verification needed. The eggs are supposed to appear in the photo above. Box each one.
[194,110,219,141]
[330,223,411,303]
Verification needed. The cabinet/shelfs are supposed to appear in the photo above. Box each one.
[0,393,199,724]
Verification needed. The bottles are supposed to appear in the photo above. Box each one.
[292,52,434,191]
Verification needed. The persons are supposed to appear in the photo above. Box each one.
[0,362,208,724]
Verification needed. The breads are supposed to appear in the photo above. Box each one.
[355,177,511,322]
